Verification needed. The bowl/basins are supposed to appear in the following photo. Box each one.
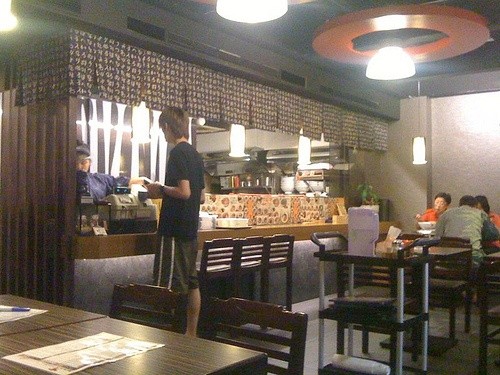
[295,181,324,193]
[281,177,295,194]
[418,222,436,230]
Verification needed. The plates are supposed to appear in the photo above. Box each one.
[216,226,252,229]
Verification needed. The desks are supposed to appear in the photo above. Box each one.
[0,291,269,375]
[380,245,471,358]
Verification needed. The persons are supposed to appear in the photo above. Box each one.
[435,195,500,281]
[76,140,153,205]
[475,195,500,248]
[415,192,451,230]
[144,105,206,337]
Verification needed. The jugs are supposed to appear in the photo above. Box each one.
[348,208,379,256]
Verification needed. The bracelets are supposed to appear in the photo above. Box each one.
[160,186,164,195]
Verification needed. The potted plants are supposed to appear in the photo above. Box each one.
[356,183,381,222]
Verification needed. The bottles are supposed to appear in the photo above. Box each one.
[257,177,260,187]
[115,172,129,193]
[266,176,270,186]
[392,240,414,258]
[231,175,240,188]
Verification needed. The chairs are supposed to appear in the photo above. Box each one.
[430,236,473,338]
[205,296,310,375]
[107,281,189,334]
[476,251,500,375]
[196,233,298,308]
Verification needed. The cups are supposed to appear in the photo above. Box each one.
[218,217,249,227]
[242,183,246,187]
[248,181,254,187]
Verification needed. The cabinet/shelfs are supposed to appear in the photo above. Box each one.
[310,232,435,375]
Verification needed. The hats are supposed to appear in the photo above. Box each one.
[77,145,92,163]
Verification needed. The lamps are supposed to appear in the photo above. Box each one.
[215,0,289,24]
[366,38,417,81]
[0,0,18,32]
[229,123,247,158]
[412,78,427,166]
[297,135,312,166]
